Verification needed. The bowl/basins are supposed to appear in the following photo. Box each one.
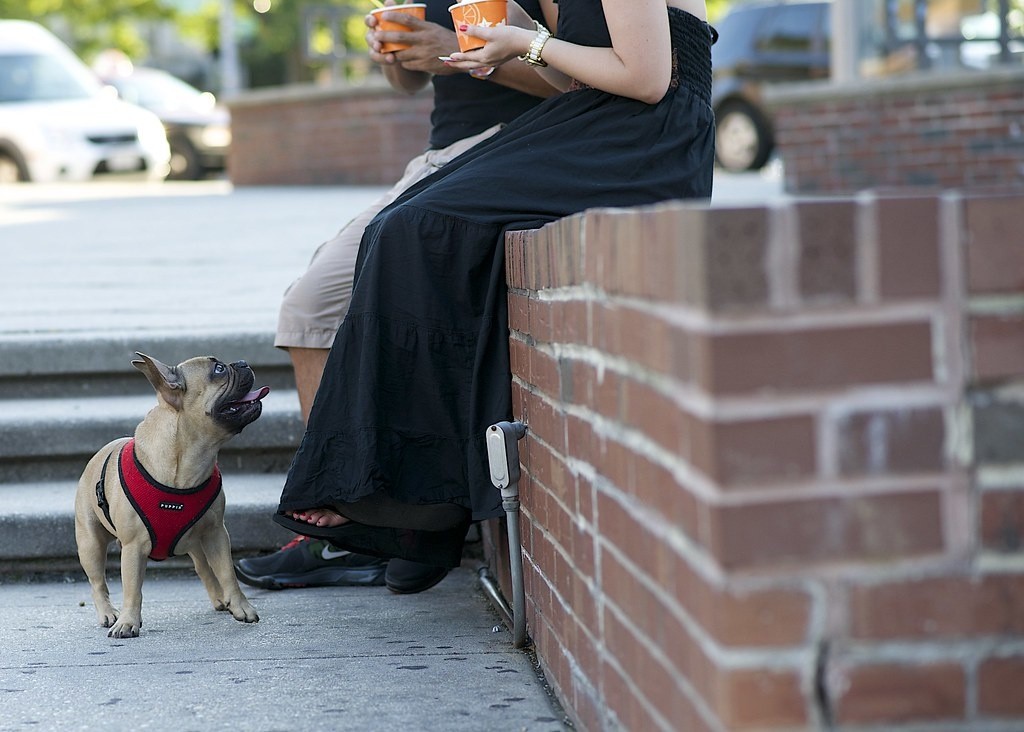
[448,0,508,53]
[370,3,427,54]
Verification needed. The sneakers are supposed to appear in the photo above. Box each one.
[233,535,391,590]
[385,557,454,594]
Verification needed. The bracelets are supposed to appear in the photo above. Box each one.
[470,66,496,79]
[527,32,551,67]
[517,21,545,60]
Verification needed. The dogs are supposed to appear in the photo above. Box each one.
[73,349,272,640]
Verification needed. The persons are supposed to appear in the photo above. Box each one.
[231,0,713,598]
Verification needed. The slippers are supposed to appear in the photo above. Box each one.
[273,504,374,537]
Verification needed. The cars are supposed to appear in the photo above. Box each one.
[0,17,171,182]
[93,66,233,180]
[711,2,1021,175]
